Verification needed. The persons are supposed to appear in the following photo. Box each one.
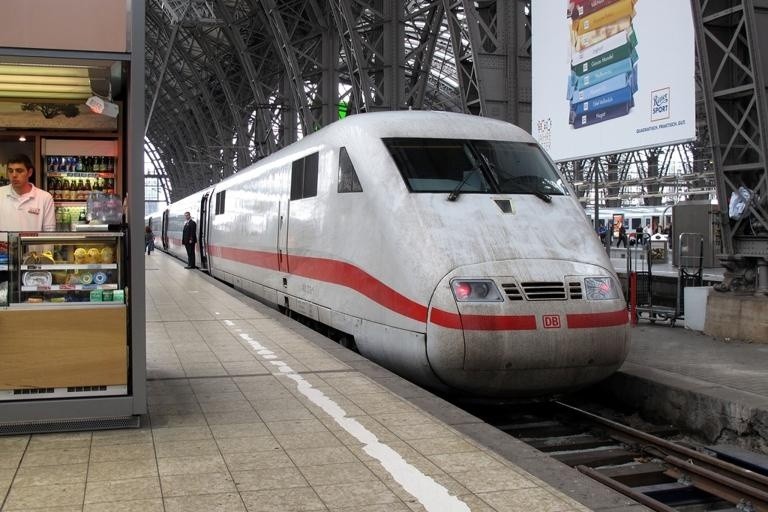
[145,224,157,255]
[182,211,199,269]
[592,221,673,249]
[0,152,58,302]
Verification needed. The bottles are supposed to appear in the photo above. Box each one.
[47,156,123,231]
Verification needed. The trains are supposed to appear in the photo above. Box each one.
[582,204,673,253]
[145,107,631,411]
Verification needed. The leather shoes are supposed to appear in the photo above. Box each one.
[185,266,194,269]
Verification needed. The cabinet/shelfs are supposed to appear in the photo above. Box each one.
[31,132,123,223]
[0,231,129,393]
